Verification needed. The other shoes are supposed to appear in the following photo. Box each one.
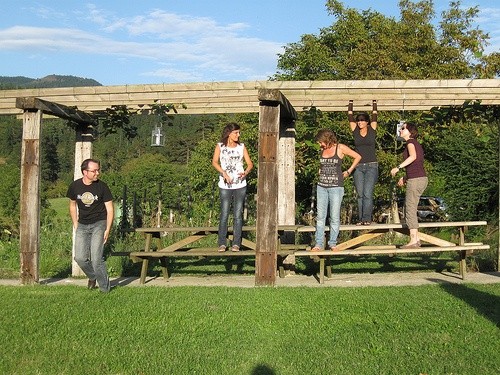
[88,279,96,290]
[312,246,325,252]
[231,245,240,252]
[356,221,371,226]
[217,245,227,252]
[399,241,421,249]
[330,247,338,252]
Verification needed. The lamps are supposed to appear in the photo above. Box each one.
[150,121,164,147]
[396,102,406,140]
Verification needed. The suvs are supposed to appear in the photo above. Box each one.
[374,195,449,226]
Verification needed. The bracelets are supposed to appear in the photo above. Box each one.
[397,165,401,170]
[347,170,349,174]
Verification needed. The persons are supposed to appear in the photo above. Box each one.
[391,121,429,248]
[348,100,380,225]
[210,123,253,251]
[309,129,361,252]
[66,159,114,293]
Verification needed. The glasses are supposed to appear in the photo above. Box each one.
[402,128,407,130]
[85,169,99,173]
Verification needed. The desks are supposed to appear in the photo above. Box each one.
[120,225,305,284]
[300,220,488,284]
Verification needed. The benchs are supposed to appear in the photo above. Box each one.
[111,245,308,257]
[295,242,491,256]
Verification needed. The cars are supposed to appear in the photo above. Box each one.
[298,194,317,217]
